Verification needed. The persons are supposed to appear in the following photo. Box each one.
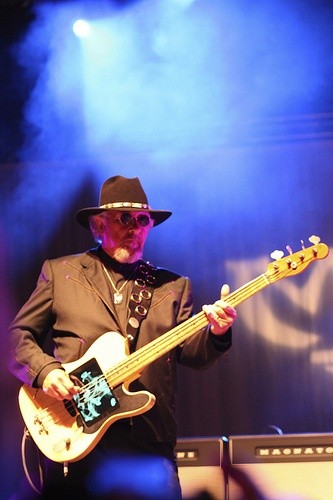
[0,175,238,500]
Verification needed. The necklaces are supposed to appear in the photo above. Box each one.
[99,259,139,306]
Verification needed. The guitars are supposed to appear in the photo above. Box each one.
[17,234,329,464]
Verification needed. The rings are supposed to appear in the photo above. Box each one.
[214,315,219,320]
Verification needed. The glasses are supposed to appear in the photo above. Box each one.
[104,211,154,227]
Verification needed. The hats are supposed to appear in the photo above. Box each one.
[75,175,173,226]
